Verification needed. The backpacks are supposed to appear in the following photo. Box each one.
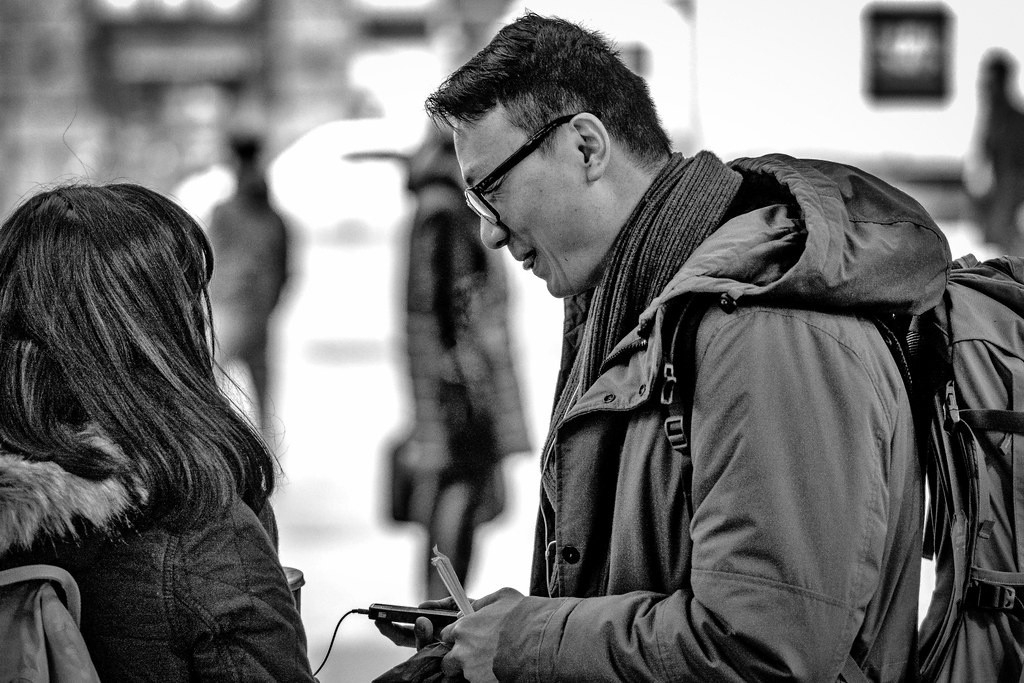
[662,252,1024,683]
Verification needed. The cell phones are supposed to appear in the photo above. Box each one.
[369,603,459,626]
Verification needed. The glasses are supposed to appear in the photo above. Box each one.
[464,113,598,224]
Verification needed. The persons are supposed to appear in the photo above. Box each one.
[951,44,1024,265]
[0,182,318,682]
[388,126,535,615]
[193,133,297,446]
[422,11,951,683]
